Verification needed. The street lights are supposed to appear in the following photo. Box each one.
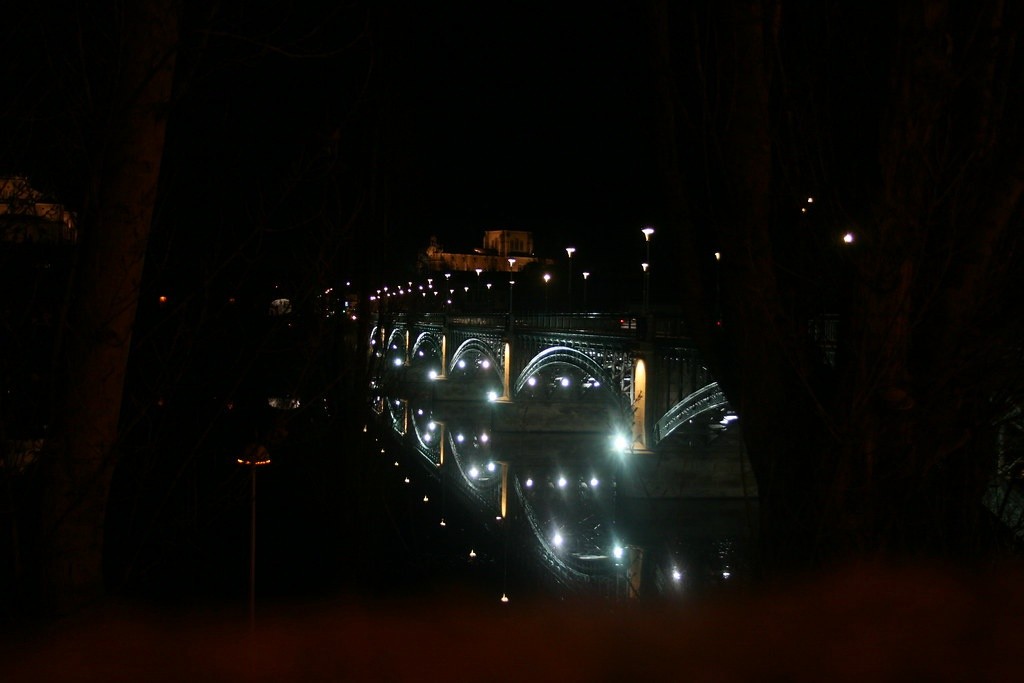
[544,275,551,313]
[641,227,654,325]
[567,247,575,313]
[509,256,515,313]
[583,272,589,313]
[445,273,451,313]
[715,253,721,308]
